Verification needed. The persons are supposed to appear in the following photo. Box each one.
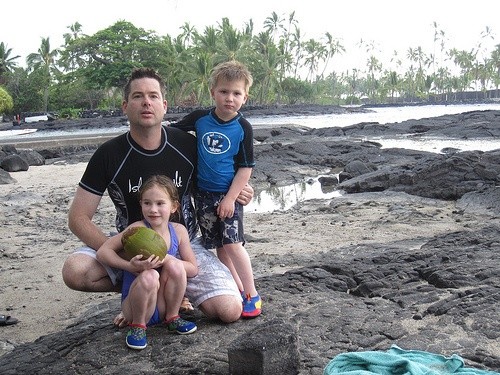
[165,60,262,317]
[95,175,200,350]
[61,68,254,327]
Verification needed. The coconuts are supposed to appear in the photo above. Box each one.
[121,226,167,261]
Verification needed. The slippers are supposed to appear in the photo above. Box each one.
[0,314,18,326]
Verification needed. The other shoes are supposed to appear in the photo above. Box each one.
[125,323,148,349]
[241,293,262,318]
[239,289,246,301]
[164,315,197,334]
